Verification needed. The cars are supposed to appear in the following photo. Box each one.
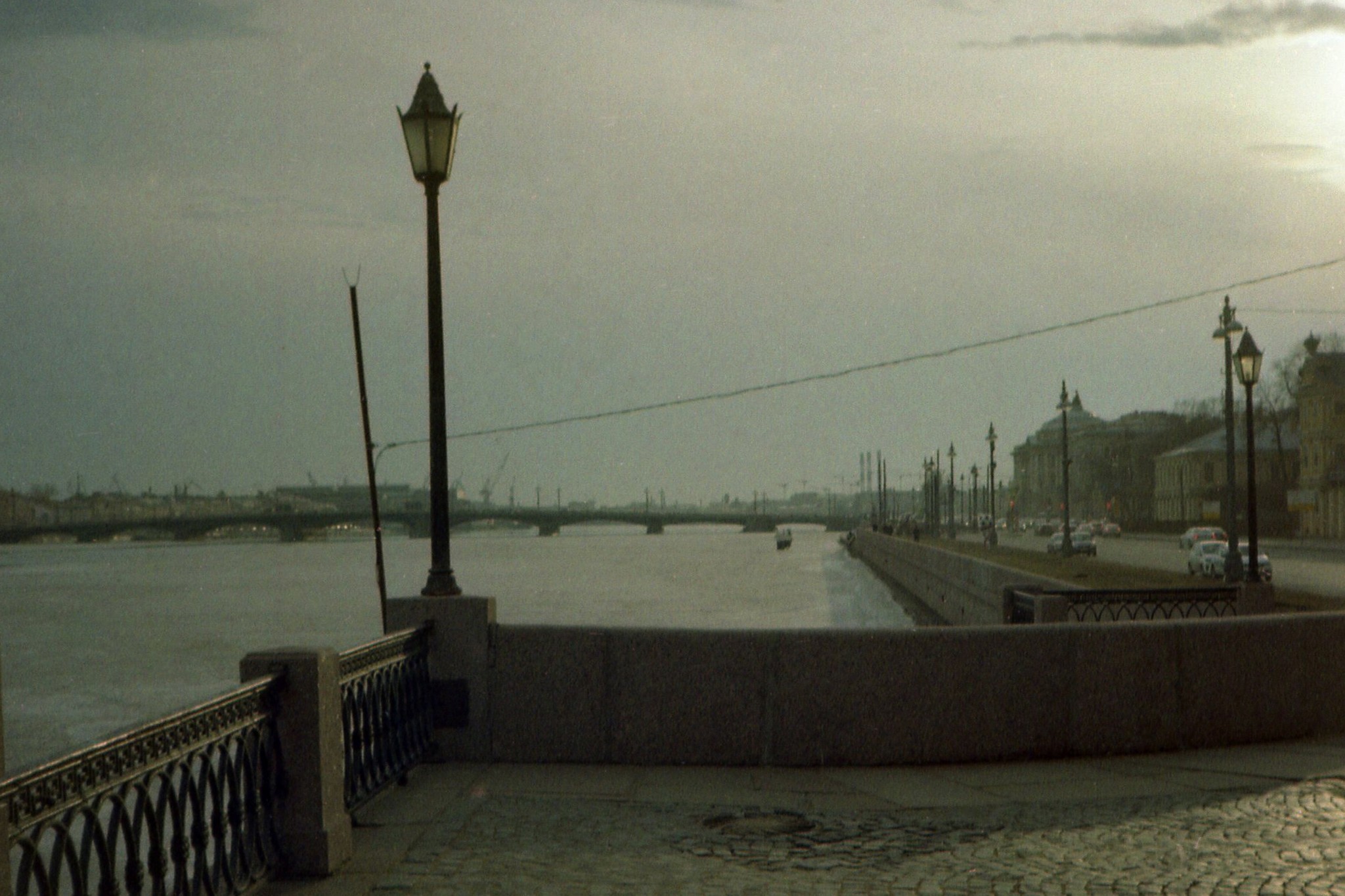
[1211,528,1230,543]
[1188,540,1224,577]
[952,514,1125,539]
[1180,527,1216,547]
[1048,533,1098,556]
[1208,542,1275,583]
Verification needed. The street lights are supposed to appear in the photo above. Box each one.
[875,422,999,543]
[1056,379,1072,558]
[1211,292,1244,588]
[391,60,467,601]
[1230,325,1267,617]
